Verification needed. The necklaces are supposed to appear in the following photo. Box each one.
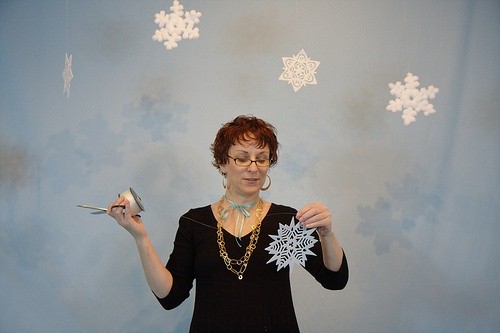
[217,195,264,276]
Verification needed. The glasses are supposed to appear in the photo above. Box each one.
[226,154,274,167]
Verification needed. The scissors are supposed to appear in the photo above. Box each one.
[74,204,142,220]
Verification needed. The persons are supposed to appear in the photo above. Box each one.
[108,114,350,333]
